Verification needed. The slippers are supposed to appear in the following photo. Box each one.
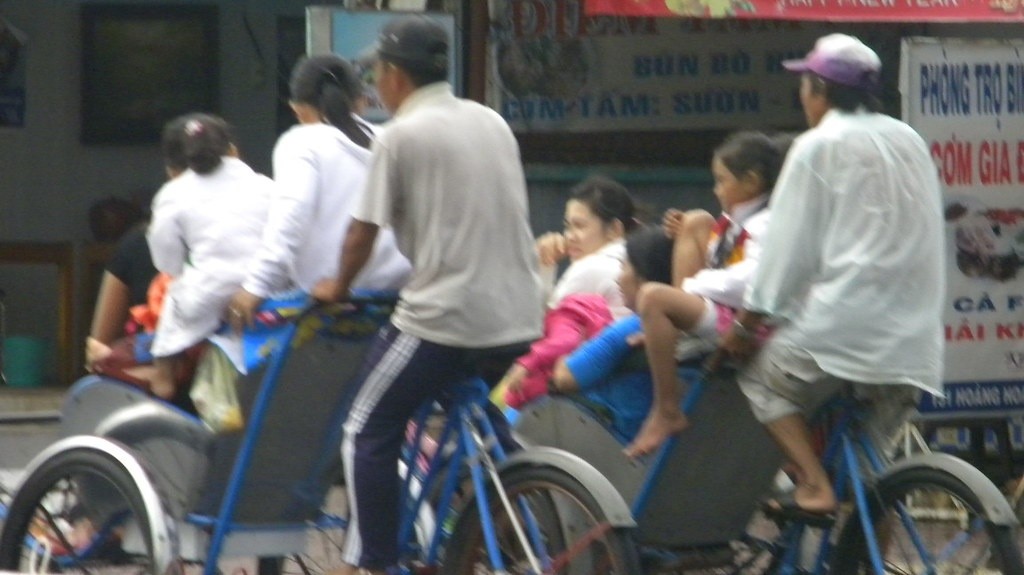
[762,491,837,529]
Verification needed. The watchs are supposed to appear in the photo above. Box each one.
[731,320,754,340]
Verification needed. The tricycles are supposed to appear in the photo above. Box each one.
[0,288,637,574]
[411,319,1024,575]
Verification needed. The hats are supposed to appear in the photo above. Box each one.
[357,10,450,70]
[783,32,881,86]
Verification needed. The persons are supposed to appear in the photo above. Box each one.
[712,32,945,575]
[81,16,787,575]
